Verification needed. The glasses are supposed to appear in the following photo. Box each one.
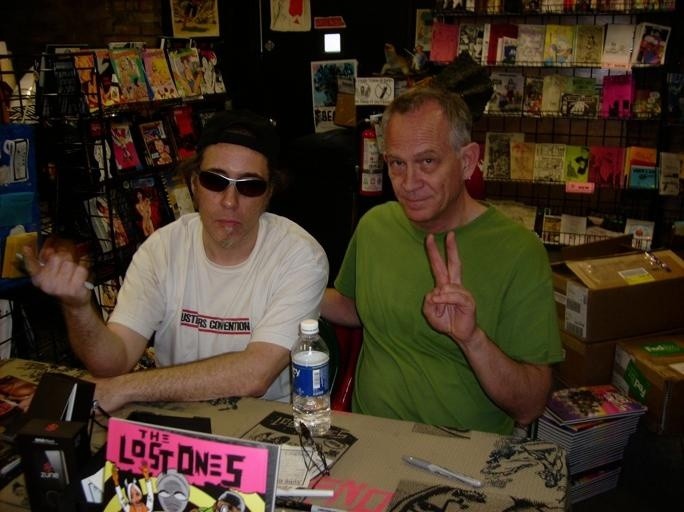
[195,169,271,198]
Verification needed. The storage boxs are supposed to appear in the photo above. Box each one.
[552,331,612,389]
[612,336,684,434]
[550,248,684,344]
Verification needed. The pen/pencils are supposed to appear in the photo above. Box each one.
[275,498,349,512]
[402,454,481,487]
[276,488,334,498]
[16,253,94,290]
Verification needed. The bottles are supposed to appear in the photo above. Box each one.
[0,42,39,125]
[291,320,332,436]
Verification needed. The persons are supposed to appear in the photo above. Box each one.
[379,37,408,74]
[19,130,330,420]
[321,87,565,436]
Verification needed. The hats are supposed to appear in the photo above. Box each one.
[182,111,283,160]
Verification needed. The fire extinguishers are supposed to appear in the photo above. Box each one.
[357,114,385,197]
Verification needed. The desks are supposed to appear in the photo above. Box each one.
[0,358,567,511]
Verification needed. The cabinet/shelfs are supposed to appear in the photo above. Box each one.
[368,1,684,249]
[0,45,237,367]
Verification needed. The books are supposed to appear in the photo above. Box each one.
[81,168,198,317]
[533,385,648,504]
[465,130,684,251]
[78,39,222,178]
[416,0,684,120]
